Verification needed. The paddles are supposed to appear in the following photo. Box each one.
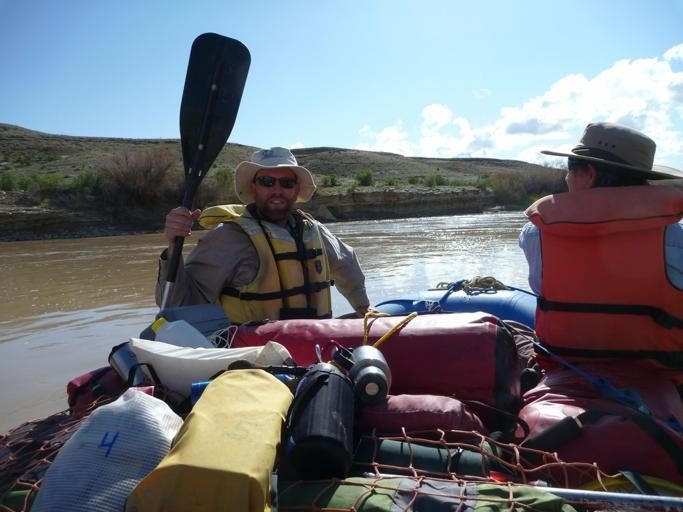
[159,32,252,312]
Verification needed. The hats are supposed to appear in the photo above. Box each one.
[235,147,316,204]
[541,122,683,180]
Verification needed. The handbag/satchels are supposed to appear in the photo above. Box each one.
[127,364,185,409]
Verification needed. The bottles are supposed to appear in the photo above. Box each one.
[151,317,216,349]
[285,345,391,482]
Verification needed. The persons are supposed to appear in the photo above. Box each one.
[156,148,377,326]
[518,124,683,361]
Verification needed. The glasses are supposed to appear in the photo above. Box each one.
[256,176,295,189]
